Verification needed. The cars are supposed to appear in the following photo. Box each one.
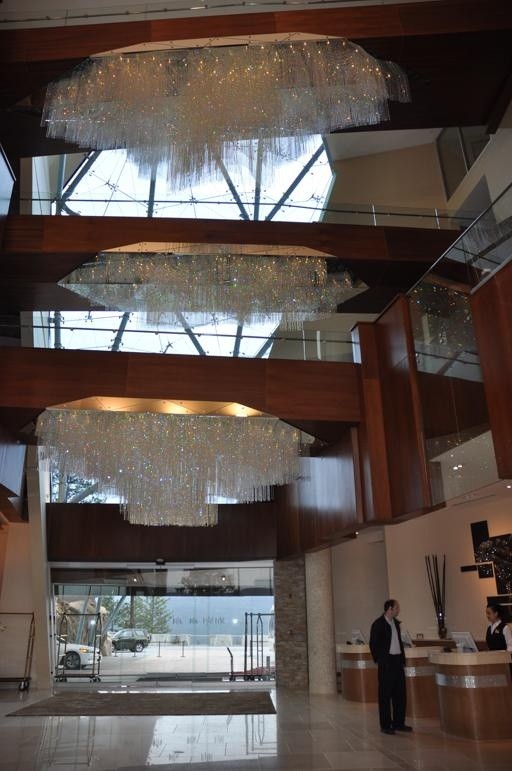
[56,635,103,668]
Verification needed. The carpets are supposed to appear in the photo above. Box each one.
[4,691,277,717]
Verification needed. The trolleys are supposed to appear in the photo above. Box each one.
[1,611,37,692]
[226,646,262,681]
[243,613,276,682]
[54,611,105,684]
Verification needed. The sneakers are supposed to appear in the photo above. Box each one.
[377,722,415,736]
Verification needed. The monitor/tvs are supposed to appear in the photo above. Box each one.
[449,631,479,653]
[351,628,367,645]
[401,631,412,647]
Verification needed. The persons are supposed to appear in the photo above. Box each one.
[368,599,414,736]
[484,602,511,653]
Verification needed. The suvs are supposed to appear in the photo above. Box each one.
[109,627,151,653]
